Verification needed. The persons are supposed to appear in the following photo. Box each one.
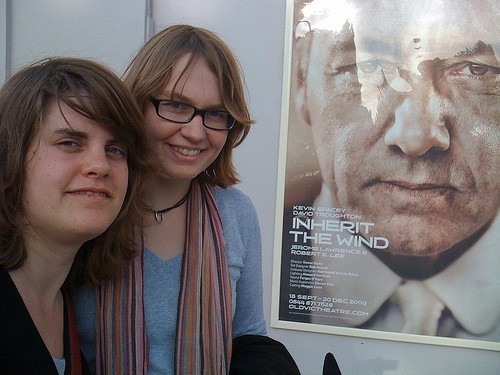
[0,55,161,374]
[64,23,270,375]
[279,1,500,342]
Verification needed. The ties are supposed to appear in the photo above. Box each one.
[389,281,447,337]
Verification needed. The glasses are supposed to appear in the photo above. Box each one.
[147,96,238,131]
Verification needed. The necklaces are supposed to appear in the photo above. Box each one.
[146,187,190,224]
